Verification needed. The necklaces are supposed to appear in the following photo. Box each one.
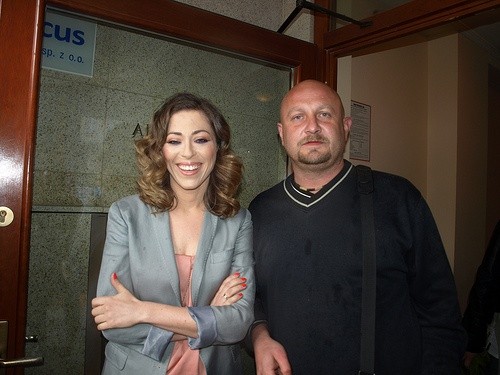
[292,178,324,192]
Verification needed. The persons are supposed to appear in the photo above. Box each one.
[90,93,258,375]
[239,79,467,375]
[463,221,499,375]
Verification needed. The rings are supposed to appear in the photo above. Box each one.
[223,294,229,300]
